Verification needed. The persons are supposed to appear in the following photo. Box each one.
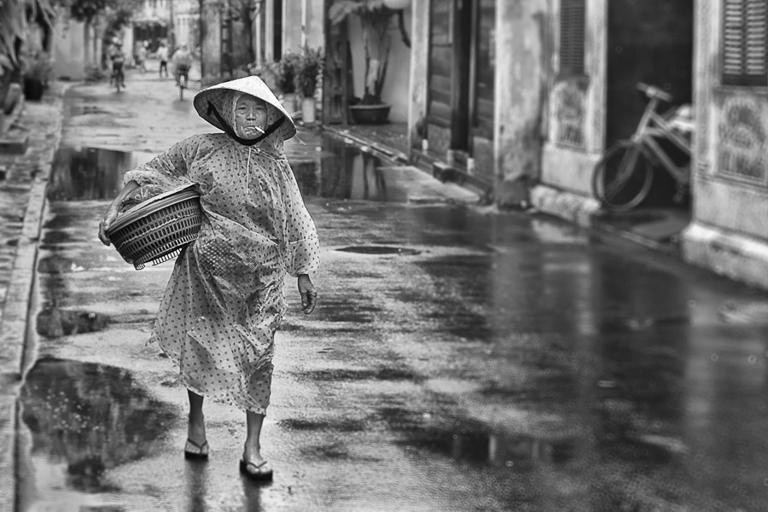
[96,74,323,482]
[105,34,194,92]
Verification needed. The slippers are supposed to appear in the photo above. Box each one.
[185,439,209,458]
[241,459,272,481]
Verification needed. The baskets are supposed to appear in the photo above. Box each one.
[105,189,201,271]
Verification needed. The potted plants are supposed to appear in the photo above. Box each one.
[22,48,56,101]
[328,0,412,126]
[296,39,329,123]
[279,48,301,115]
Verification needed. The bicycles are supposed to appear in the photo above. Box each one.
[176,68,189,101]
[591,81,695,214]
[110,54,126,92]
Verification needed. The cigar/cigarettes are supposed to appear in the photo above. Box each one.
[254,125,268,135]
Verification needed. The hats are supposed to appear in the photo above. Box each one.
[193,73,297,141]
